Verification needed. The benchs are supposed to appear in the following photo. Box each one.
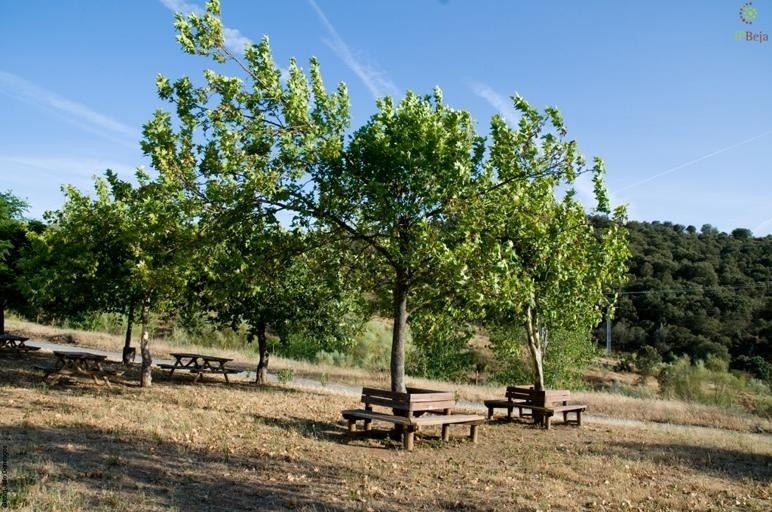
[0,333,124,390]
[191,369,238,384]
[341,384,487,452]
[158,364,210,380]
[483,385,586,429]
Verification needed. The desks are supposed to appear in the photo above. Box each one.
[168,353,234,384]
